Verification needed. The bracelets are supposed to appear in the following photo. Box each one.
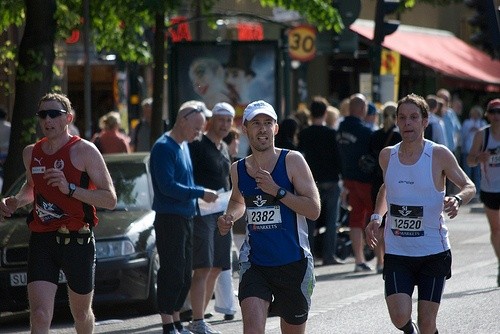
[371,214,382,223]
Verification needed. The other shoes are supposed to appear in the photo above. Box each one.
[185,320,221,334]
[353,263,370,272]
[321,256,345,266]
[162,325,195,334]
[224,312,235,320]
[376,267,384,274]
[180,311,213,322]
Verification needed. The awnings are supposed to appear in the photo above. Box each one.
[348,19,500,91]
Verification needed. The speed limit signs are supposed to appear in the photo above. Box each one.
[288,26,316,61]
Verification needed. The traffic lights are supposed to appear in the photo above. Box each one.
[128,92,142,131]
[375,1,403,40]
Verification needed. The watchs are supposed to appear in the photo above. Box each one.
[276,187,286,200]
[453,195,462,207]
[67,183,76,198]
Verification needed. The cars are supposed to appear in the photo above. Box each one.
[0,153,160,317]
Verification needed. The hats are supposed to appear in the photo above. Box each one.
[221,45,257,79]
[243,100,278,124]
[365,103,381,116]
[211,102,236,116]
[186,100,214,118]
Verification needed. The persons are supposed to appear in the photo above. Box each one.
[425,89,486,194]
[363,102,403,147]
[149,101,219,334]
[187,103,237,334]
[468,99,500,287]
[68,98,153,153]
[214,129,241,321]
[338,94,383,273]
[219,101,321,334]
[275,98,345,265]
[365,94,476,334]
[0,94,118,334]
[0,107,11,164]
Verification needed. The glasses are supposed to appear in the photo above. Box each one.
[34,110,68,120]
[486,108,500,114]
[184,107,203,119]
